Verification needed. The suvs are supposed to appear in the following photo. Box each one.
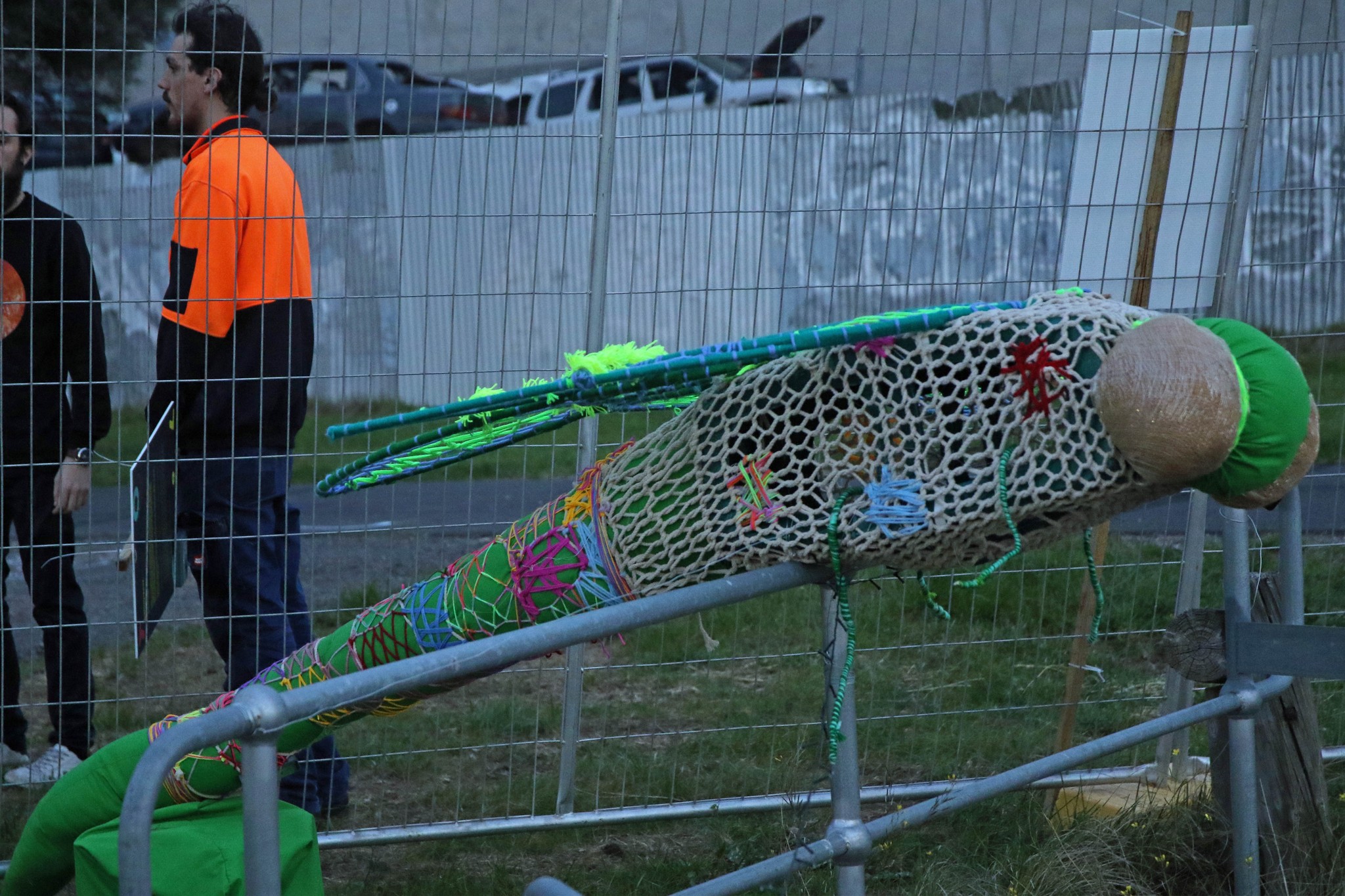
[524,51,849,120]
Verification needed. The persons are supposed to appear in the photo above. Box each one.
[0,95,115,786]
[143,0,355,823]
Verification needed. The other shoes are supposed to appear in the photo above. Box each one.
[299,790,349,819]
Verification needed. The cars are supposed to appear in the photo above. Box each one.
[14,82,114,169]
[113,51,509,163]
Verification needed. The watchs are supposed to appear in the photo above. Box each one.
[61,444,93,464]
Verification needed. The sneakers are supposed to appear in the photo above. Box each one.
[4,744,82,787]
[2,743,29,765]
